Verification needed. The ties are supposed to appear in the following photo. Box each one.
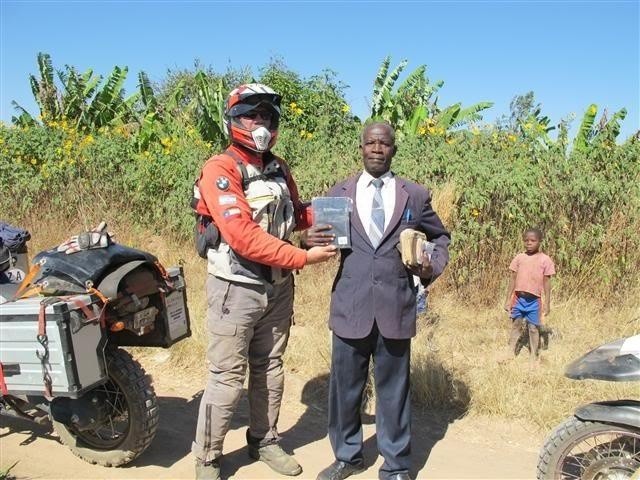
[368,180,386,250]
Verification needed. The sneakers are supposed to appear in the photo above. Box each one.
[248,435,305,478]
[189,457,225,480]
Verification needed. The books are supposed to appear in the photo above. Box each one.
[396,229,436,270]
[309,195,353,250]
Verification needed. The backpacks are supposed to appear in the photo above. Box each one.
[193,147,250,260]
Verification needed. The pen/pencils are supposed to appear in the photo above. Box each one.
[406,208,411,224]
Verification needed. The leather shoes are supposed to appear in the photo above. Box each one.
[314,458,368,480]
[378,466,412,480]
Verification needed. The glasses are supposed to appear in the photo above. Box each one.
[240,108,276,123]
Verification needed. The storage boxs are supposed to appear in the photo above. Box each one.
[110,265,192,348]
[0,290,111,401]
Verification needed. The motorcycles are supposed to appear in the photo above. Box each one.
[535,329,640,480]
[0,223,194,475]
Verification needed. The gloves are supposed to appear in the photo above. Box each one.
[56,219,114,255]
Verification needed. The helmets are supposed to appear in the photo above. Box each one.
[221,81,284,154]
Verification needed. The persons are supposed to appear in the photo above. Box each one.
[187,81,338,479]
[504,228,555,360]
[296,121,452,479]
